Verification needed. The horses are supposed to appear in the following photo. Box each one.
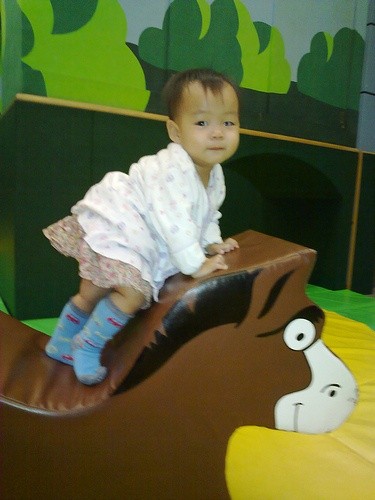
[0,231,356,499]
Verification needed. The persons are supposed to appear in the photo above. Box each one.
[40,66,241,386]
